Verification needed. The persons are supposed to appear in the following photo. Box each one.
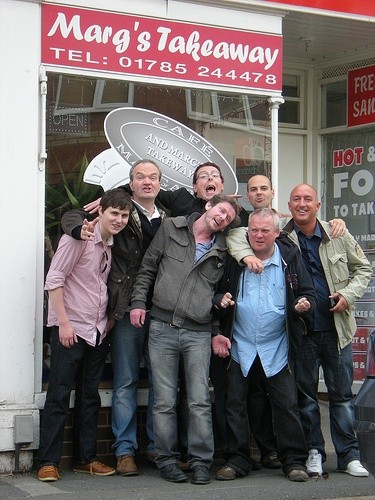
[223,170,347,473]
[61,159,243,477]
[83,161,250,472]
[225,183,375,477]
[211,208,319,482]
[35,187,133,480]
[129,194,240,486]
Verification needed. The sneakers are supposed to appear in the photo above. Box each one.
[73,457,116,476]
[37,466,61,481]
[305,449,323,475]
[344,460,368,476]
[116,455,139,475]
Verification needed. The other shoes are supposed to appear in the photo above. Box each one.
[261,450,282,468]
[216,466,236,480]
[287,469,308,481]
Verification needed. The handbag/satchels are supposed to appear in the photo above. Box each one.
[105,269,129,315]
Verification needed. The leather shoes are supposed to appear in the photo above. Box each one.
[161,463,188,482]
[192,465,211,484]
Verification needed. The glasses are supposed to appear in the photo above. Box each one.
[194,170,220,179]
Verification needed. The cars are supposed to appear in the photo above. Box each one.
[350,330,374,477]
